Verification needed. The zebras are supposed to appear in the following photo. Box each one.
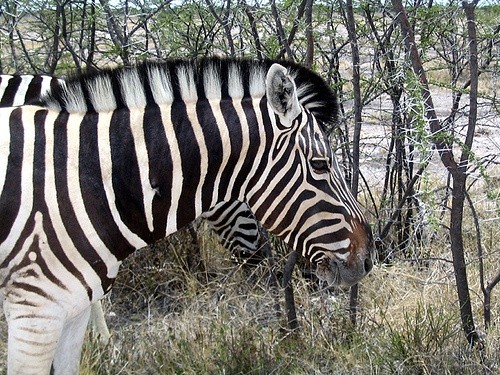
[0,57,372,375]
[0,72,273,375]
